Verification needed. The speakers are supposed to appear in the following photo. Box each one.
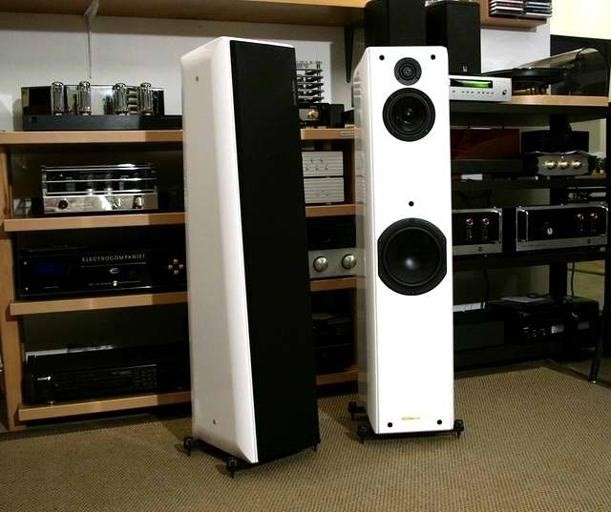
[351,44,457,434]
[363,0,426,46]
[426,0,481,75]
[179,36,320,460]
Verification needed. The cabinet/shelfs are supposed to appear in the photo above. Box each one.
[450,96,611,381]
[0,129,359,434]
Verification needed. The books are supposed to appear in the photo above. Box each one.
[490,0,552,19]
